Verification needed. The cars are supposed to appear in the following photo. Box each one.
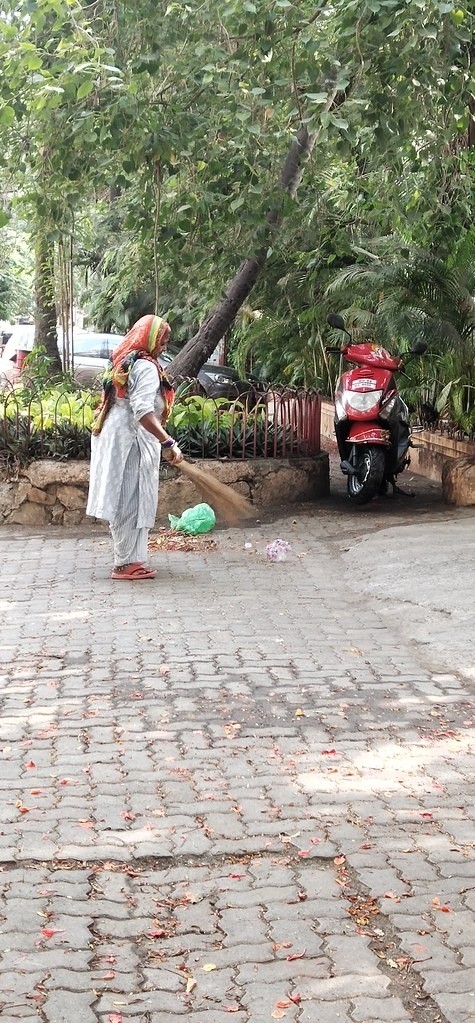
[57,333,264,415]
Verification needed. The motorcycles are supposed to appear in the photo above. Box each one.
[323,313,428,505]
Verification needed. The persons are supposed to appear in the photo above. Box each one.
[86,315,182,579]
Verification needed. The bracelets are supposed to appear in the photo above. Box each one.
[160,437,176,448]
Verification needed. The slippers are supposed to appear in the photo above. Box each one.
[111,563,155,579]
